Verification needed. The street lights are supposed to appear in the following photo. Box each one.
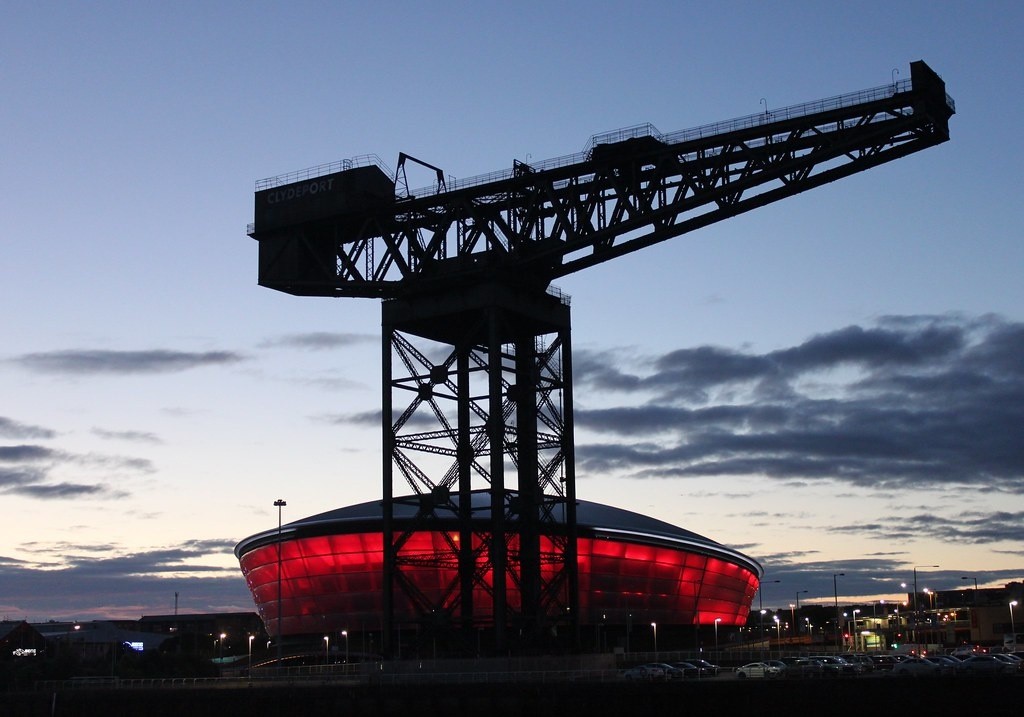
[1009,600,1018,651]
[772,614,780,656]
[796,591,808,625]
[804,618,809,635]
[759,580,781,642]
[342,630,348,673]
[248,636,255,681]
[650,622,657,653]
[852,609,860,652]
[834,573,844,616]
[961,577,977,591]
[913,564,939,611]
[714,617,721,665]
[323,636,329,674]
[273,498,287,668]
[789,604,796,637]
[219,633,227,680]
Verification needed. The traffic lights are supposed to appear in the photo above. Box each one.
[898,633,901,639]
[844,632,848,641]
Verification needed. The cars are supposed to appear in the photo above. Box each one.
[666,661,705,677]
[644,662,681,677]
[761,660,787,676]
[623,664,666,683]
[778,645,1024,682]
[736,661,781,680]
[685,659,721,676]
